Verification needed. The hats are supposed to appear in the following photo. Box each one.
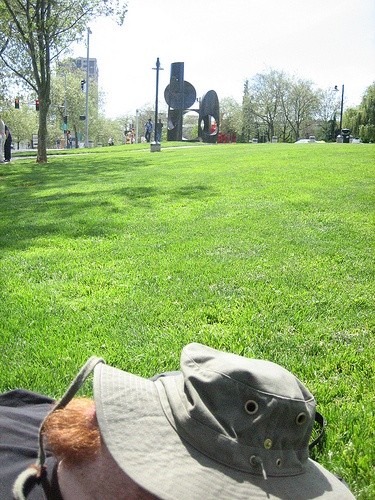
[91,342,355,500]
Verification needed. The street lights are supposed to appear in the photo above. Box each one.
[85,26,93,147]
[332,85,344,134]
[60,73,67,148]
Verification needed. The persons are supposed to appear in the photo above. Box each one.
[108,137,115,146]
[131,128,135,144]
[144,118,153,142]
[0,343,357,500]
[69,136,73,149]
[5,125,12,162]
[0,115,7,163]
[57,137,61,149]
[155,119,163,142]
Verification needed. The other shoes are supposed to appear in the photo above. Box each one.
[4,159,10,162]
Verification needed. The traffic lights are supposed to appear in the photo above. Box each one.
[15,99,19,108]
[80,115,85,120]
[63,115,67,124]
[36,100,39,111]
[81,80,85,90]
